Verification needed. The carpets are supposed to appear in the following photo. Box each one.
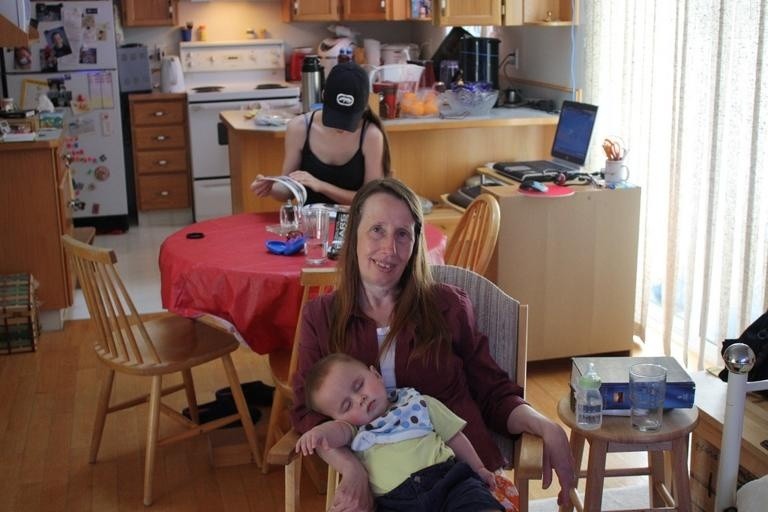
[527,483,650,512]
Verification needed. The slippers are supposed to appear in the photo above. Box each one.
[215,381,277,407]
[181,399,262,430]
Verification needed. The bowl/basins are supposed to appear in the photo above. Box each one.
[446,90,499,113]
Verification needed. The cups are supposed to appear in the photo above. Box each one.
[258,29,265,38]
[199,26,207,40]
[300,207,330,265]
[604,160,630,183]
[181,29,192,41]
[246,28,255,39]
[628,363,667,432]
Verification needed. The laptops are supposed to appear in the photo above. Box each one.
[494,100,598,182]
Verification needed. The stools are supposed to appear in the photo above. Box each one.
[557,396,699,512]
[73,226,96,289]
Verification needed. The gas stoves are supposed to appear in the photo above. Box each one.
[179,39,300,103]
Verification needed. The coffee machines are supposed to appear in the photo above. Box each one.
[457,37,502,108]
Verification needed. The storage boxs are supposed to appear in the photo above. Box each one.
[117,46,152,93]
[569,357,696,417]
[0,273,41,355]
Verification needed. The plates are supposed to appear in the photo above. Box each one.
[293,47,313,53]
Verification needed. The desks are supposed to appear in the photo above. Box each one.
[688,367,768,512]
[441,162,641,363]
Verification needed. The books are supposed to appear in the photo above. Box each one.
[2,126,63,143]
[256,175,350,220]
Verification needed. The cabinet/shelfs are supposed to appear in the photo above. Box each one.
[0,107,74,333]
[279,0,341,23]
[341,0,410,22]
[119,0,179,27]
[504,0,578,28]
[433,0,503,27]
[128,92,193,227]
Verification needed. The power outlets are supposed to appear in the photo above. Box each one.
[505,49,519,70]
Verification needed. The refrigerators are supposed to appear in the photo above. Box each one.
[0,1,131,234]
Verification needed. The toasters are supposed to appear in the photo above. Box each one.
[380,43,421,65]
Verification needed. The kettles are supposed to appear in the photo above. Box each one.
[160,55,186,94]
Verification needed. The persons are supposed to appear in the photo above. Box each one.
[51,32,71,57]
[290,177,579,511]
[250,62,392,204]
[294,350,506,510]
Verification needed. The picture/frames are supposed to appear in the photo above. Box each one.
[18,79,48,111]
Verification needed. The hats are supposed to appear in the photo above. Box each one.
[322,62,370,132]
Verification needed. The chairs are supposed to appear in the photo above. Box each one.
[61,234,263,507]
[441,194,501,275]
[261,267,363,494]
[266,265,544,511]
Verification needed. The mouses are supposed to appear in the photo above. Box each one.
[519,180,548,192]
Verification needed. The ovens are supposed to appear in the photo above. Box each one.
[187,98,303,224]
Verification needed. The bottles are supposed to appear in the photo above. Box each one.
[338,48,348,64]
[419,0,427,18]
[279,196,299,233]
[345,50,352,63]
[301,55,325,114]
[576,363,603,430]
